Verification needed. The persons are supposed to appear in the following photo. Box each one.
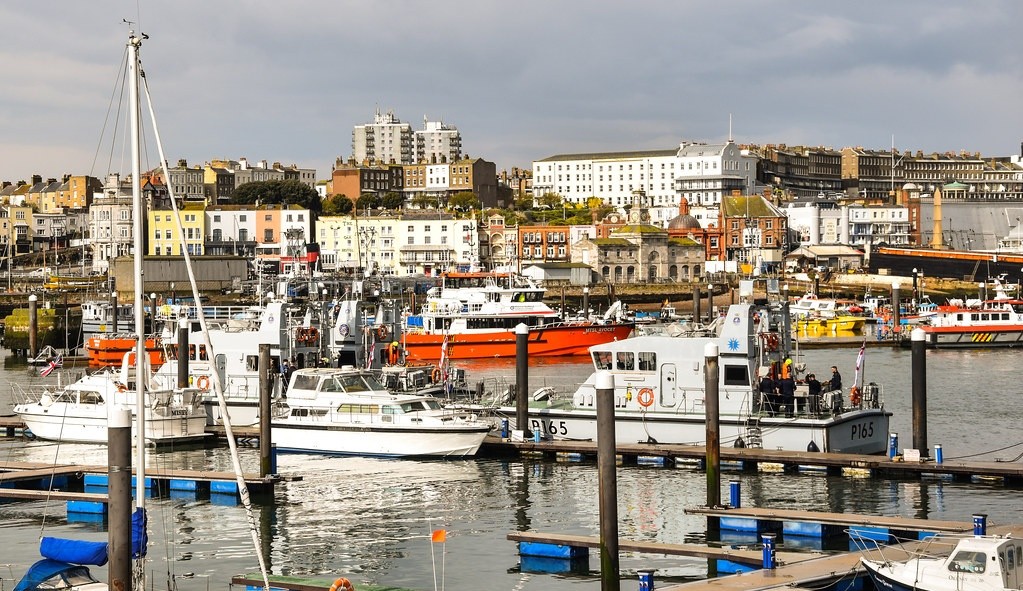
[639,358,642,366]
[515,291,524,301]
[779,373,796,418]
[760,373,778,417]
[280,359,297,377]
[606,359,612,369]
[829,366,841,390]
[805,372,821,413]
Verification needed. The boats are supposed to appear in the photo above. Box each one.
[869,210,1023,350]
[495,225,894,457]
[844,528,1023,591]
[392,218,634,367]
[139,271,492,460]
[7,342,207,442]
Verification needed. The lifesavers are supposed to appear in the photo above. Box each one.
[850,387,860,404]
[638,388,655,407]
[768,331,779,351]
[328,577,354,591]
[377,324,388,339]
[197,376,209,391]
[296,327,318,342]
[431,368,441,385]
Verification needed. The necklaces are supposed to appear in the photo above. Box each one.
[774,373,783,387]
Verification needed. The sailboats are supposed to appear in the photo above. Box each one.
[12,15,451,591]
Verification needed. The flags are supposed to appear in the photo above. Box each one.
[39,358,61,377]
[851,349,865,388]
[439,341,448,367]
[368,342,375,369]
